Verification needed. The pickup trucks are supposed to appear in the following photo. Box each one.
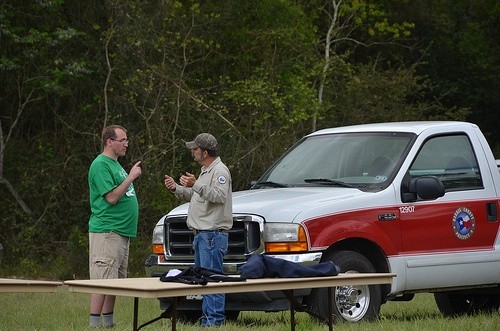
[145,122,500,326]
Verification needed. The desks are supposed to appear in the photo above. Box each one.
[64,272,397,331]
[0,278,62,293]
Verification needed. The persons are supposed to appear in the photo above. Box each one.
[87,125,141,328]
[164,133,233,327]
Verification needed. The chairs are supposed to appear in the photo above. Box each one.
[444,157,474,189]
[368,156,392,176]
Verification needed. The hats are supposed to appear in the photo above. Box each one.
[186,133,218,150]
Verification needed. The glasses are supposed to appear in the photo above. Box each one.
[110,138,129,145]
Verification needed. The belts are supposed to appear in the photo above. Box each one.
[193,228,228,235]
